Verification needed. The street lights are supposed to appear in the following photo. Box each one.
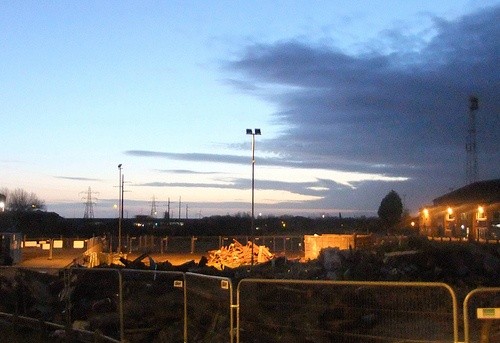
[246,128,262,268]
